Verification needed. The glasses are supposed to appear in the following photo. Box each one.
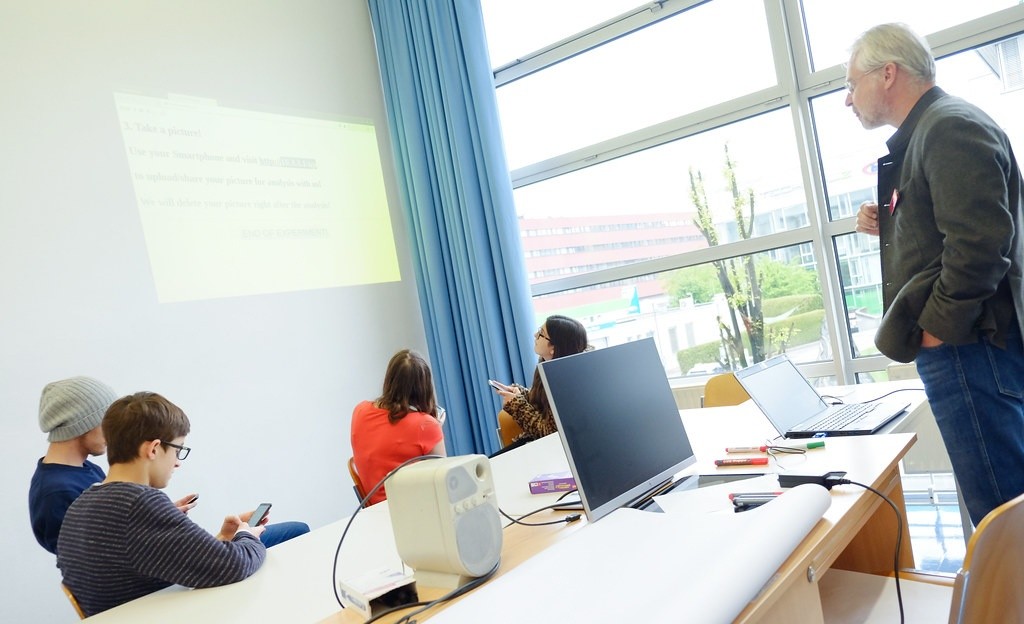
[159,441,192,460]
[536,327,554,345]
[842,64,886,93]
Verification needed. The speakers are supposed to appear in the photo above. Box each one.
[384,453,503,590]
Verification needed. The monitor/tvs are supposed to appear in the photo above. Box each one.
[536,337,697,524]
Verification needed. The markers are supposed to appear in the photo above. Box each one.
[715,458,768,465]
[729,492,783,513]
[726,441,825,452]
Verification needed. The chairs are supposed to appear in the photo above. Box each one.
[816,493,1024,624]
[700,372,751,408]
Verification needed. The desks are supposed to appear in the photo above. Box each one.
[80,380,928,623]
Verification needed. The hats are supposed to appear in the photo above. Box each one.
[37,377,121,443]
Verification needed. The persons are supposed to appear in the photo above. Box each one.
[57,390,270,618]
[350,349,447,506]
[495,315,587,458]
[28,375,310,555]
[846,25,1024,528]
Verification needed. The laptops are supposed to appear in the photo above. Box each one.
[734,354,912,439]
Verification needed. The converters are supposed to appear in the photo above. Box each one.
[779,469,832,491]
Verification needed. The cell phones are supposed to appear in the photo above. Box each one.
[185,496,198,505]
[247,502,271,528]
[488,380,506,391]
[436,406,445,419]
